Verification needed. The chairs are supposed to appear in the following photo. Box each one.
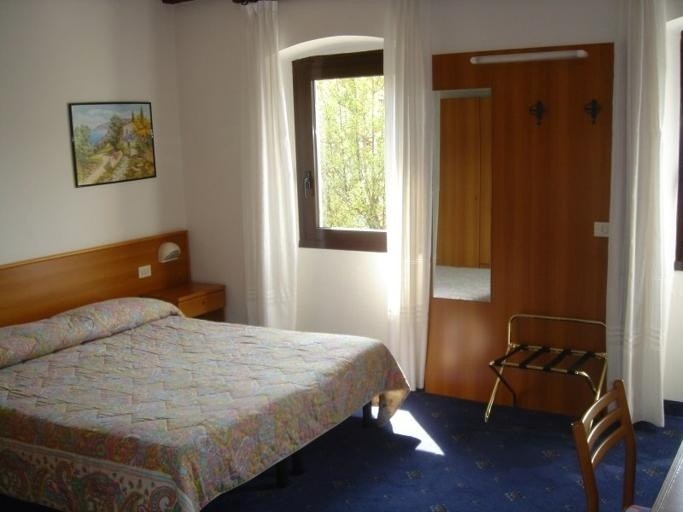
[568,379,656,512]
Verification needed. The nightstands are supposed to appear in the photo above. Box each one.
[141,282,226,322]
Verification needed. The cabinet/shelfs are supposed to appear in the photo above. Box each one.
[436,96,492,268]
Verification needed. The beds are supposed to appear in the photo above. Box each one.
[1,230,385,512]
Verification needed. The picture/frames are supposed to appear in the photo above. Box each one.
[69,101,156,186]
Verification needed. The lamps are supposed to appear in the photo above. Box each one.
[158,242,181,264]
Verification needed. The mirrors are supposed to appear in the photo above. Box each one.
[431,88,494,303]
